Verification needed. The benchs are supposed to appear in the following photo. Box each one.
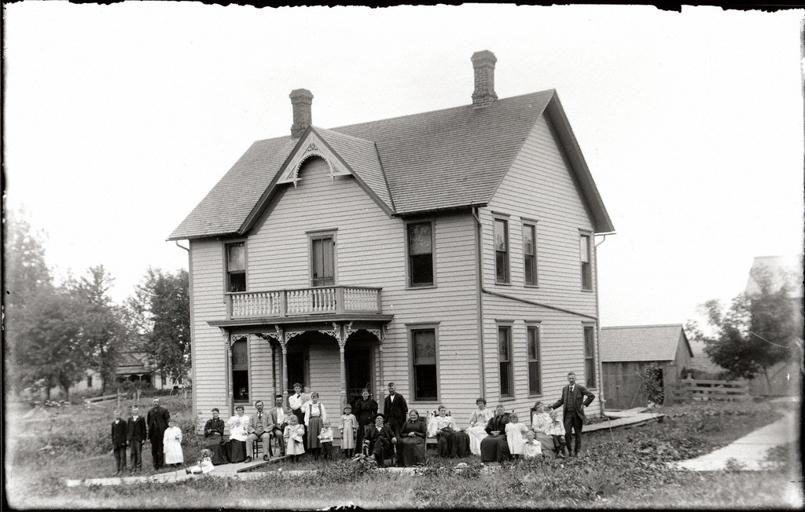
[425,411,451,460]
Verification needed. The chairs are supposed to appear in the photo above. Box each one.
[405,411,428,427]
[530,406,568,455]
[362,424,396,466]
[494,409,519,462]
[253,438,273,460]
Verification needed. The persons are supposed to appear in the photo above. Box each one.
[398,406,457,466]
[109,398,184,475]
[549,372,595,456]
[354,382,408,466]
[283,383,327,464]
[234,385,248,401]
[204,395,292,465]
[317,420,333,459]
[340,405,360,457]
[464,398,567,458]
